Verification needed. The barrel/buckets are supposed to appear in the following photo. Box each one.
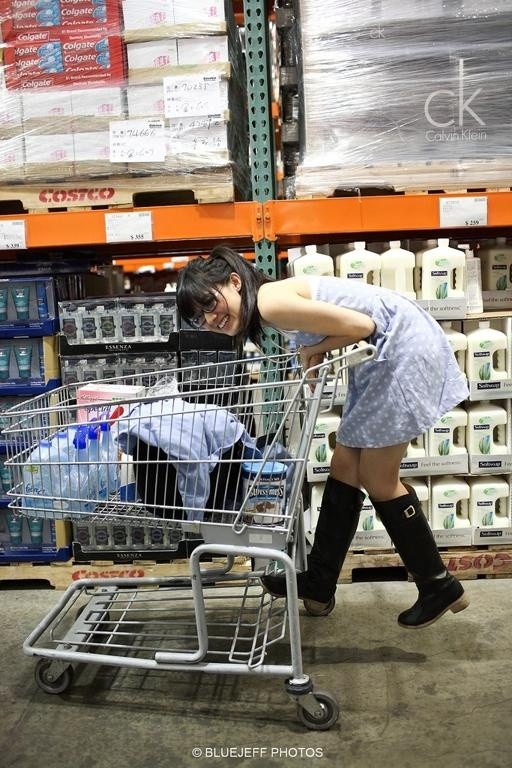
[291,241,511,533]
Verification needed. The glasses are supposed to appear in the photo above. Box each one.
[190,285,223,327]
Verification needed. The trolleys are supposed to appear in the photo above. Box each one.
[0,339,378,731]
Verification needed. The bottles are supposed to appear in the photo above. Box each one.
[68,421,121,520]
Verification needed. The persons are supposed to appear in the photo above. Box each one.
[172,242,472,632]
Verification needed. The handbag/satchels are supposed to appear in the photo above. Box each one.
[131,437,243,519]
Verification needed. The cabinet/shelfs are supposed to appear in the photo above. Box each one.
[0,0,512,591]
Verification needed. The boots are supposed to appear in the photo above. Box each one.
[260,475,365,614]
[367,481,470,630]
